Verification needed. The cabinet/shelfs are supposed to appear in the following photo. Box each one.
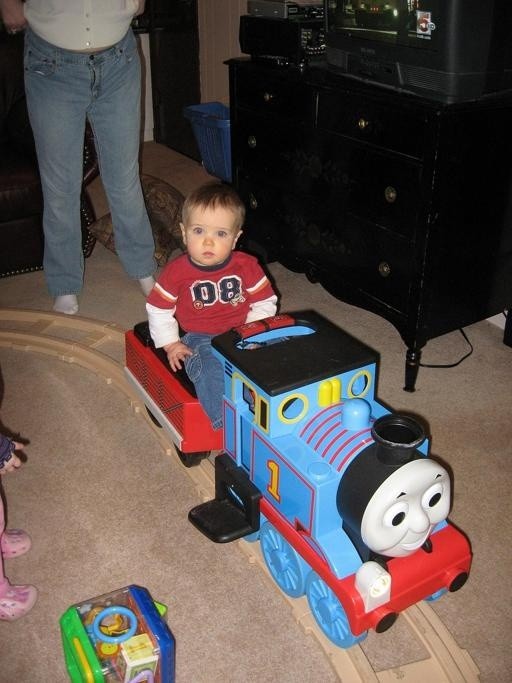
[225,56,512,392]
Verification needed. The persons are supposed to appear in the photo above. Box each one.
[145,178,279,430]
[0,433,39,621]
[0,0,158,315]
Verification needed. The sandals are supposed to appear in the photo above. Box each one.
[1,527,33,560]
[0,583,40,624]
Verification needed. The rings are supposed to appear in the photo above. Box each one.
[11,28,17,34]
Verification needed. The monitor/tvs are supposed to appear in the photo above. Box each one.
[323,0,512,105]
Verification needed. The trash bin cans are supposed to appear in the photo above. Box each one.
[183,101,232,184]
